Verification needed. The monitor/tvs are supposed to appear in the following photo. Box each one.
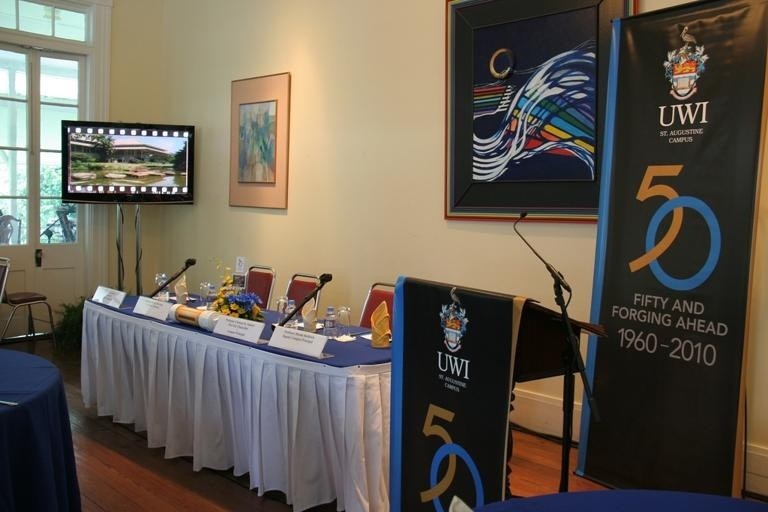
[61,119,195,206]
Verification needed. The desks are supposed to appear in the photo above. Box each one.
[81,294,393,512]
[455,489,768,512]
[1,347,82,512]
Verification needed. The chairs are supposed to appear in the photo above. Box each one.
[244,266,276,311]
[286,272,320,316]
[359,280,395,338]
[2,288,57,355]
[0,256,11,304]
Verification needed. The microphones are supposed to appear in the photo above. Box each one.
[513,209,572,294]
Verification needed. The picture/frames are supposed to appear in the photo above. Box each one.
[230,72,291,209]
[444,0,640,224]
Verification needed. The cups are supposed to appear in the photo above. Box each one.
[337,306,351,340]
[275,296,288,328]
[198,280,210,311]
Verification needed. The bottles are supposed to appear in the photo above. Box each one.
[324,307,336,339]
[154,272,169,303]
[207,285,218,310]
[284,299,298,330]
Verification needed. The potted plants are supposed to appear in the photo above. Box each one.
[54,296,89,362]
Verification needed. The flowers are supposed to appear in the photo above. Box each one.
[206,265,266,322]
